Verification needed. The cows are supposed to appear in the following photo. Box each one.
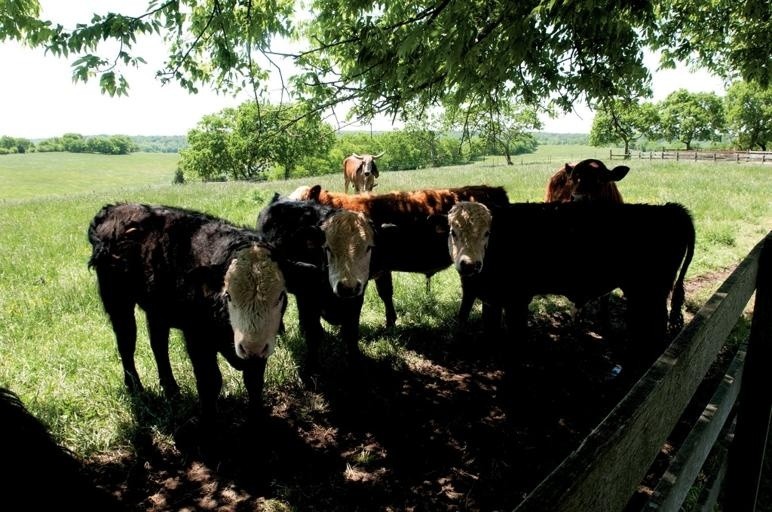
[547,159,630,203]
[427,200,696,370]
[256,192,397,368]
[288,185,508,329]
[342,151,387,194]
[87,200,317,428]
